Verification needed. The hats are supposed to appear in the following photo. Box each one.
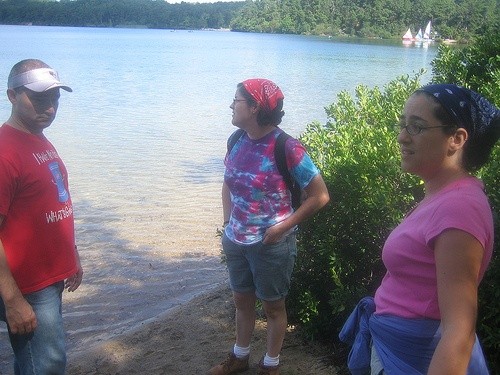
[7,68,72,94]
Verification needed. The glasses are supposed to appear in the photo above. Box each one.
[393,124,447,137]
[233,99,248,102]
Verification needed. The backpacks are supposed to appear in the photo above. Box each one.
[227,129,308,211]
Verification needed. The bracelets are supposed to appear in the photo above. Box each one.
[75,245,77,249]
[224,221,229,225]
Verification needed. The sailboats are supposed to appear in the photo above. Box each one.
[423,21,431,39]
[415,28,423,39]
[402,28,414,40]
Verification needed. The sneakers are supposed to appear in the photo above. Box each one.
[256,358,280,375]
[208,353,250,375]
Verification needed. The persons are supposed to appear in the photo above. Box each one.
[0,57,82,375]
[366,82,500,375]
[210,77,329,375]
